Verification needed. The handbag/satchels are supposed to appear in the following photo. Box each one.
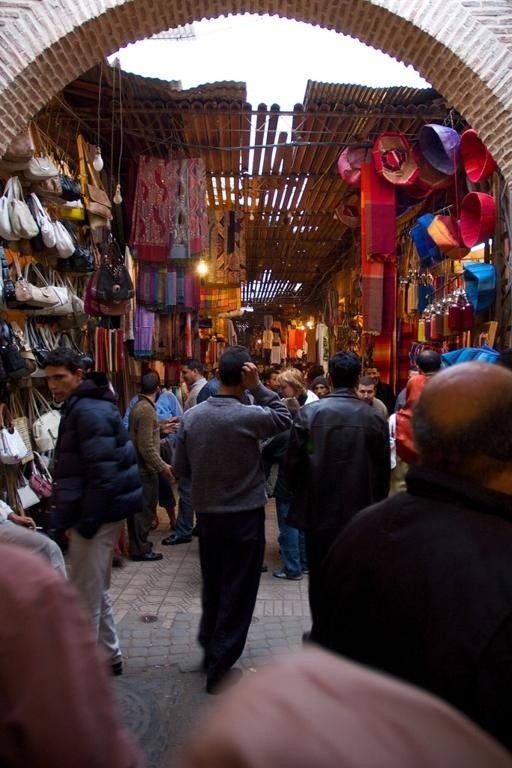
[0,317,94,385]
[387,407,423,471]
[0,126,135,317]
[0,386,62,466]
[2,450,54,509]
[417,287,474,343]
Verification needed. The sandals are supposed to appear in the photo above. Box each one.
[151,517,159,529]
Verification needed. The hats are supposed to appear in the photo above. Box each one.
[372,129,422,189]
[309,376,328,390]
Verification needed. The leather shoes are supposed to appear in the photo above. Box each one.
[206,667,243,695]
[131,551,163,561]
[161,533,192,546]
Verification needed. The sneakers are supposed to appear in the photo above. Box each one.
[110,661,124,676]
[273,568,309,581]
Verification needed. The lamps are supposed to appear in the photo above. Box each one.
[92,60,105,173]
[112,63,125,205]
